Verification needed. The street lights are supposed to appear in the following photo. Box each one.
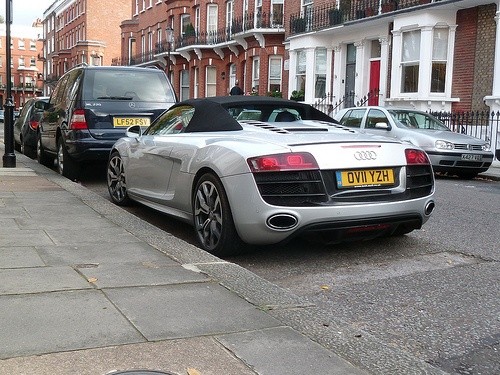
[165,25,174,80]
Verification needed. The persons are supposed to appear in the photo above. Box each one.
[230,80,243,95]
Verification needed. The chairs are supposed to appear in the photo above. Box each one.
[274,112,295,122]
[371,112,388,126]
[345,117,358,125]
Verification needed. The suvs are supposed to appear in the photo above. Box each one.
[36,62,181,179]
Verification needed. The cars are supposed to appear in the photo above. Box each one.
[0,110,4,122]
[13,96,53,155]
[331,105,493,176]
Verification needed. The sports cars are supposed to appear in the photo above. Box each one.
[107,94,436,252]
